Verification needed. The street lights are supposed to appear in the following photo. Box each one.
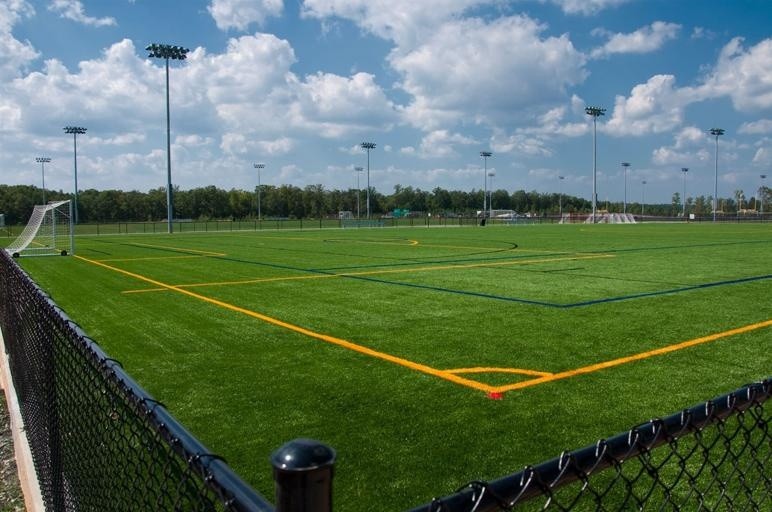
[478,151,492,220]
[708,128,725,219]
[488,173,495,219]
[36,158,50,225]
[558,176,567,213]
[361,143,376,219]
[253,163,266,220]
[759,174,767,211]
[145,43,189,234]
[682,167,689,215]
[355,167,364,219]
[584,107,607,224]
[641,181,648,213]
[620,162,630,213]
[62,126,87,225]
